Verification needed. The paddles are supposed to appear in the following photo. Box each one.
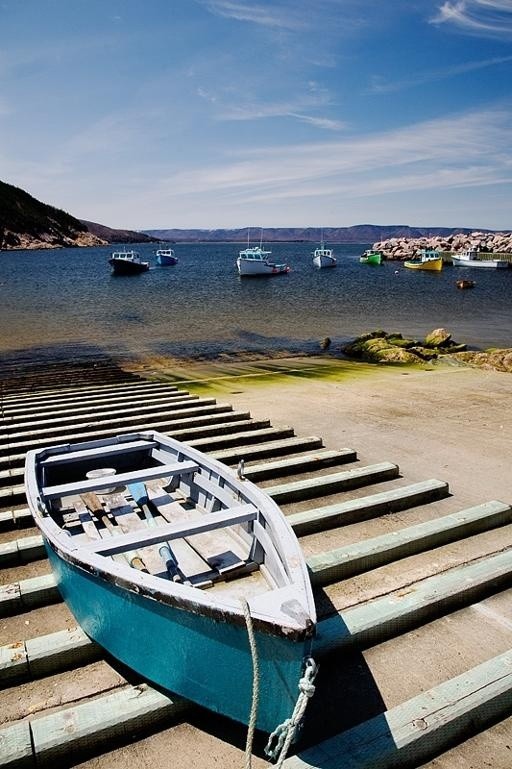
[80,492,150,574]
[128,483,182,582]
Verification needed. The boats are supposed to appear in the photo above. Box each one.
[25,428,318,748]
[109,246,149,273]
[311,240,336,268]
[456,279,476,290]
[236,229,289,276]
[403,250,443,272]
[450,249,508,269]
[360,249,383,265]
[156,248,178,266]
